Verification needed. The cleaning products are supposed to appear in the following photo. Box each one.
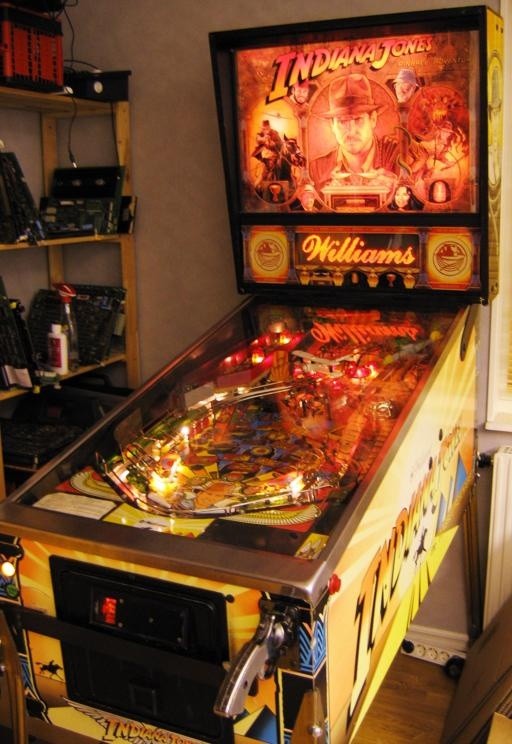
[52,281,78,367]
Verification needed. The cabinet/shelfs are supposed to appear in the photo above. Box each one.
[2,84,139,442]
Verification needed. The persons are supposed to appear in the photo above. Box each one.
[305,73,401,207]
[251,120,284,180]
[389,72,422,103]
